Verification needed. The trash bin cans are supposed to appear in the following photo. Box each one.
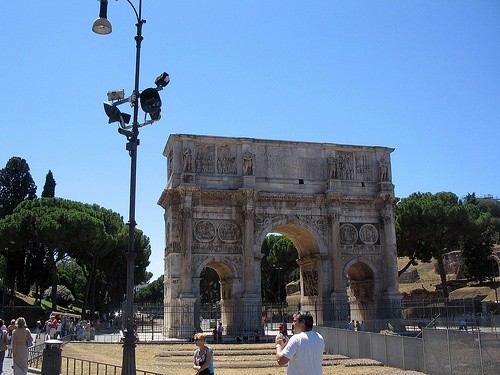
[41,340,64,375]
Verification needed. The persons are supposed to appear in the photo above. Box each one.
[11,317,33,375]
[275,310,324,375]
[1,318,17,358]
[34,319,42,343]
[0,319,8,375]
[45,315,90,341]
[213,323,223,343]
[254,328,260,342]
[192,333,214,375]
[348,320,365,331]
[242,328,248,342]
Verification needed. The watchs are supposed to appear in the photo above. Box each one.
[275,344,280,348]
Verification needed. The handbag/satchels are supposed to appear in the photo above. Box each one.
[198,361,210,375]
[26,329,33,347]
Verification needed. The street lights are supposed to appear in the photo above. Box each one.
[92,0,171,375]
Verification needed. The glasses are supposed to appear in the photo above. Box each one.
[194,339,200,341]
